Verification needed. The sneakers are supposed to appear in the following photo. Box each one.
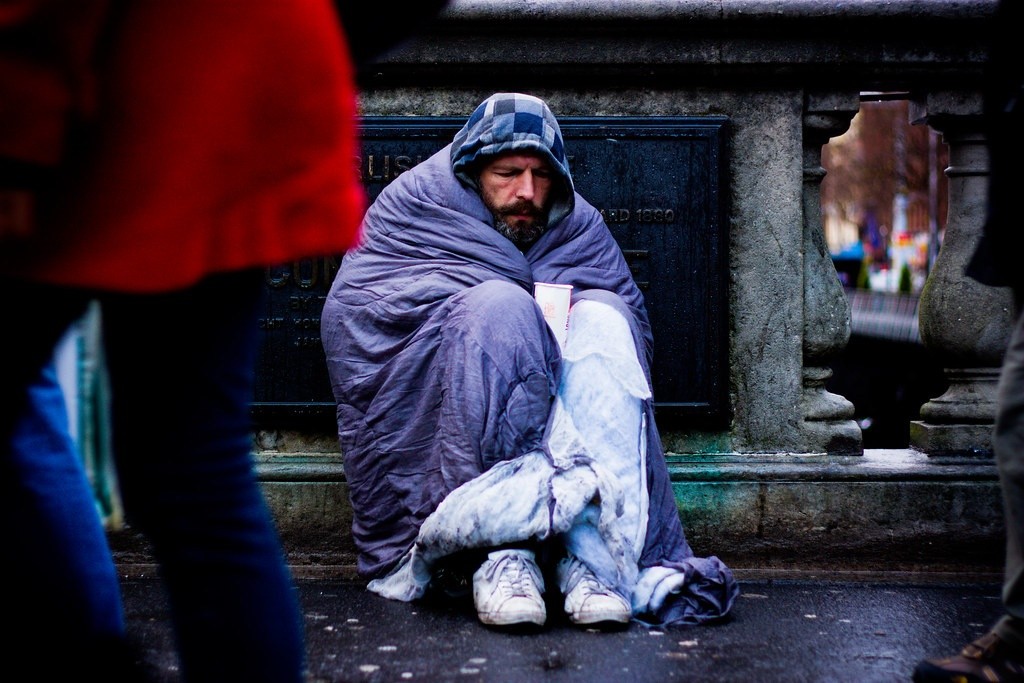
[555,549,633,626]
[472,548,547,628]
[912,614,1024,683]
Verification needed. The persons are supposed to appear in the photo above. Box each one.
[320,93,742,627]
[1,1,367,683]
[910,1,1024,683]
